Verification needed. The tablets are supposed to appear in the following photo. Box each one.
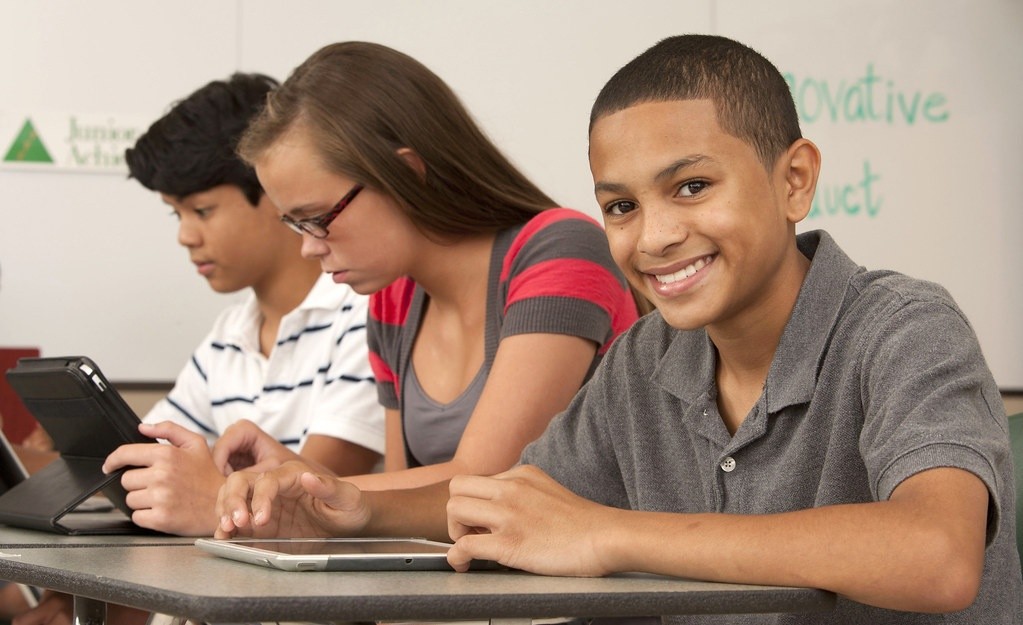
[7,356,159,520]
[194,538,484,572]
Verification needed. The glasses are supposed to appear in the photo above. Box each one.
[281,184,367,237]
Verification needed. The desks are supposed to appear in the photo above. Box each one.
[0,524,839,625]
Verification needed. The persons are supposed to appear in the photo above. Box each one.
[211,35,1023,625]
[101,40,657,537]
[20,71,388,480]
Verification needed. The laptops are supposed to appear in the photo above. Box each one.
[0,432,114,514]
